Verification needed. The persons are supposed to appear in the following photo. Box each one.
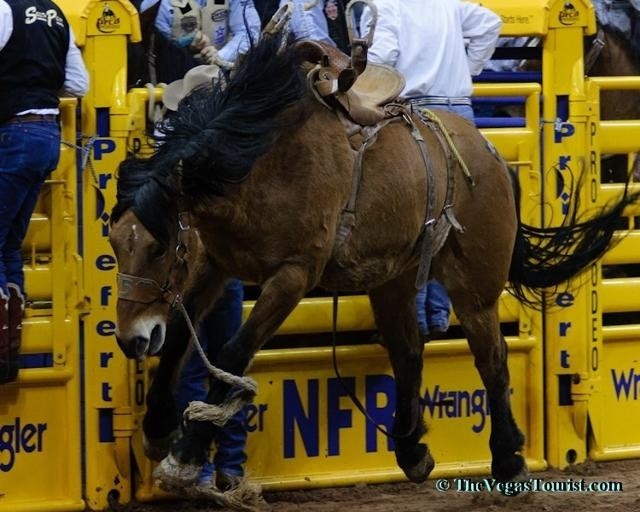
[346,0,504,351]
[128,2,548,134]
[141,60,274,511]
[1,1,88,386]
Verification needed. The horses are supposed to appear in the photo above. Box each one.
[130,0,208,94]
[492,0,640,182]
[107,0,640,492]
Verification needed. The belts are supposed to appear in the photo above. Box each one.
[409,97,472,105]
[12,112,60,123]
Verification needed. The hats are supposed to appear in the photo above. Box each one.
[159,61,229,111]
